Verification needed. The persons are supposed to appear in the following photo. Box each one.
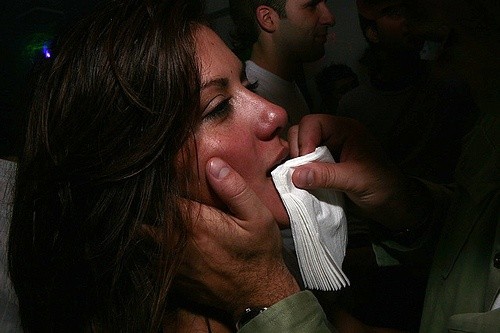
[7,0,500,333]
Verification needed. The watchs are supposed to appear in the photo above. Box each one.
[236,307,266,329]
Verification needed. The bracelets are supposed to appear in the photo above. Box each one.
[385,215,431,246]
